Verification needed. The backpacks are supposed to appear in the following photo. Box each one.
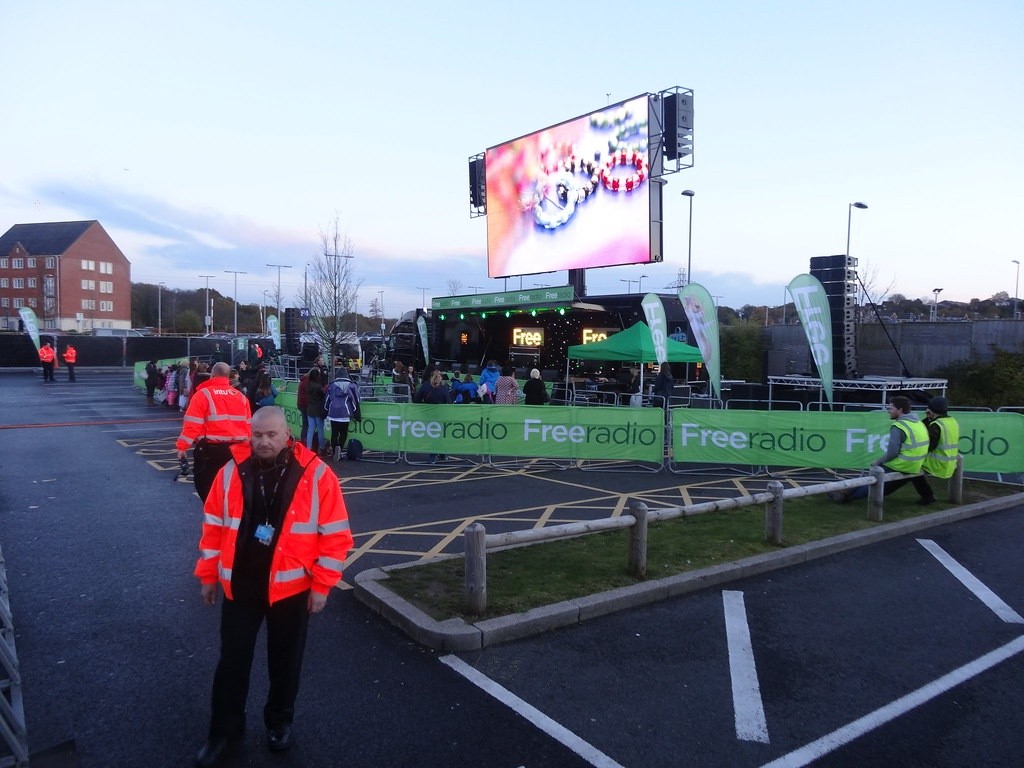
[347,439,363,460]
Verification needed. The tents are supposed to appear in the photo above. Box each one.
[565,321,712,409]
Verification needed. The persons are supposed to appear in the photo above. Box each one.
[296,353,361,461]
[39,343,57,382]
[62,343,76,381]
[193,407,352,768]
[176,362,252,504]
[392,361,546,405]
[623,363,640,405]
[368,354,386,383]
[144,344,277,413]
[826,397,959,504]
[654,362,674,407]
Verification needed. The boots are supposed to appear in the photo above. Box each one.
[146,396,157,406]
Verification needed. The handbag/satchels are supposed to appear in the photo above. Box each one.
[542,389,551,403]
[179,394,189,407]
[140,364,149,380]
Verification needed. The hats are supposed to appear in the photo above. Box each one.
[336,367,348,379]
[928,397,948,414]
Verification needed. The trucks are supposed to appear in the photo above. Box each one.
[385,283,722,399]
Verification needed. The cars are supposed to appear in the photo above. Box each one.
[203,332,390,374]
[132,326,163,337]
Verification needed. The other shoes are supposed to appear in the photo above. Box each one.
[918,495,936,506]
[333,445,341,463]
[441,455,448,461]
[180,410,187,413]
[827,491,844,503]
[428,457,436,464]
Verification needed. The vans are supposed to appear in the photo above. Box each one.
[90,327,145,337]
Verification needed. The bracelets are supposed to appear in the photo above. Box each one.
[511,100,649,229]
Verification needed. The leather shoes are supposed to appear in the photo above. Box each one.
[268,721,291,750]
[196,733,238,768]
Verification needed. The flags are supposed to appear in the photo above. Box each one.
[19,307,39,351]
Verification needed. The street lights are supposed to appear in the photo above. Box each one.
[158,281,166,338]
[264,289,269,333]
[377,290,386,337]
[682,188,695,284]
[620,279,639,294]
[224,270,247,333]
[467,286,483,294]
[710,294,725,319]
[198,276,215,333]
[416,286,432,310]
[639,275,649,292]
[847,201,870,256]
[534,283,551,289]
[1013,259,1021,318]
[265,263,292,333]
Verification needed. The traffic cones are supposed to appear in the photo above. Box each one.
[324,254,355,332]
